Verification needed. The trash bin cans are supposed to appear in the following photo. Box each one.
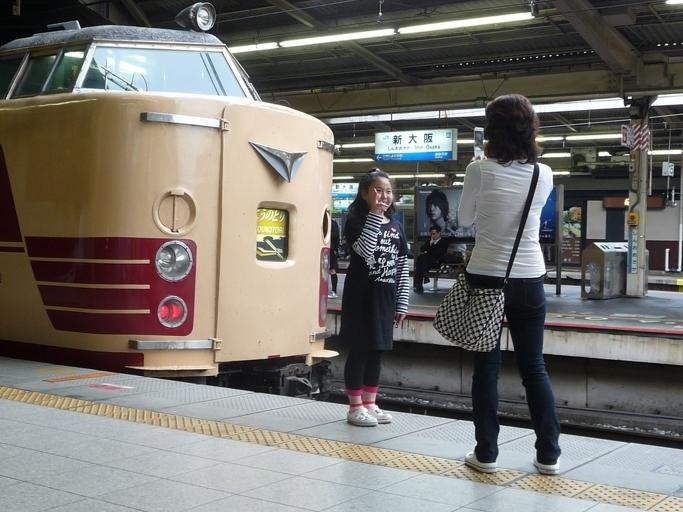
[581,241,649,301]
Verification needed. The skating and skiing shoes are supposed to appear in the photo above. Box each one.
[464,450,497,475]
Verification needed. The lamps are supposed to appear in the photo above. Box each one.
[220,2,541,57]
[308,84,682,187]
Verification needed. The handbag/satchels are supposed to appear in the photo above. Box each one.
[431,271,505,353]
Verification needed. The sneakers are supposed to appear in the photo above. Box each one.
[327,291,339,299]
[532,457,561,475]
[346,408,379,427]
[364,405,394,424]
[412,277,430,294]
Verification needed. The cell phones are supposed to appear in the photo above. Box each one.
[474,127,485,159]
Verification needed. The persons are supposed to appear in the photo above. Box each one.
[339,170,412,427]
[424,190,463,236]
[327,219,341,301]
[454,93,566,476]
[411,224,448,294]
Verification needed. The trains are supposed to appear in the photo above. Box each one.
[0,3,339,400]
